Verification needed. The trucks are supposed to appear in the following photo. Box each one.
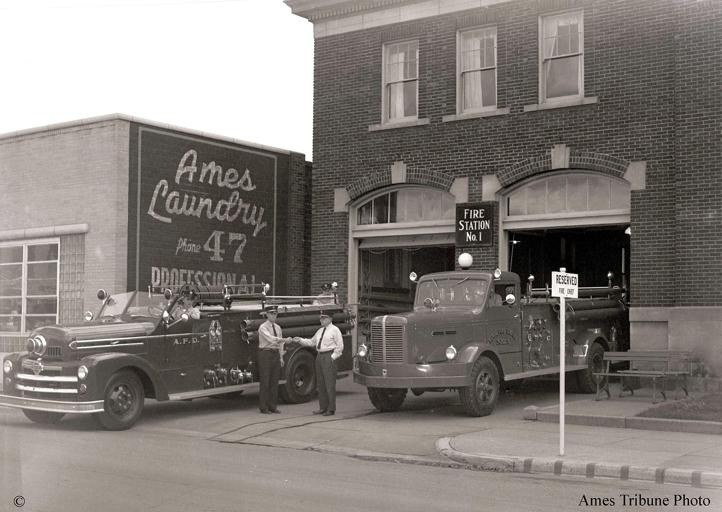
[352,270,628,416]
[2,283,351,431]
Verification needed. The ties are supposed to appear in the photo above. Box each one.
[272,324,277,336]
[318,327,326,349]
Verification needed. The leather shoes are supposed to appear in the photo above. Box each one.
[260,408,280,414]
[312,408,334,416]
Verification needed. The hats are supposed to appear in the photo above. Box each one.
[321,283,331,291]
[265,306,278,313]
[318,310,334,318]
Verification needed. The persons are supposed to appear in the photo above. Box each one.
[290,309,344,417]
[313,283,380,305]
[175,284,202,321]
[257,306,294,414]
[474,283,503,307]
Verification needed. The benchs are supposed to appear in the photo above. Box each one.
[590,349,692,404]
